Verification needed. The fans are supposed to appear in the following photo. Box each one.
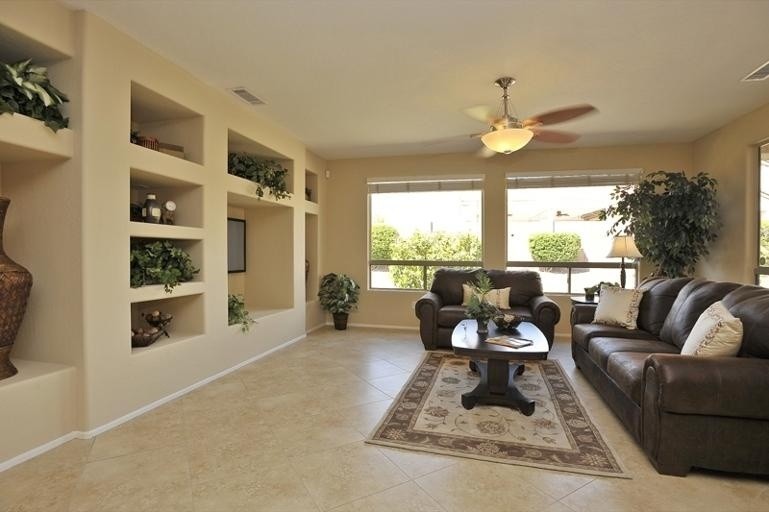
[460,77,596,159]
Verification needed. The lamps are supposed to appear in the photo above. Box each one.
[482,129,534,155]
[607,235,642,289]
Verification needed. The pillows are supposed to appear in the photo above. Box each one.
[680,301,743,358]
[593,285,643,329]
[463,285,512,309]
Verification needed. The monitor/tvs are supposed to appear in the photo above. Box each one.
[227,217,246,274]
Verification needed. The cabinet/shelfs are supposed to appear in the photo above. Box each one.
[0,0,328,466]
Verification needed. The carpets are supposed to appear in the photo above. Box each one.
[365,349,634,480]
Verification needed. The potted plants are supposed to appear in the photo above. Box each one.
[319,273,359,330]
[467,269,497,334]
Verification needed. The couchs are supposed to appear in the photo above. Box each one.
[568,276,769,477]
[415,269,561,352]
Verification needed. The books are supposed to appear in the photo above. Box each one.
[158,142,185,152]
[158,148,184,159]
[483,335,535,349]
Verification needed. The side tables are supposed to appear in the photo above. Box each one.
[570,296,598,324]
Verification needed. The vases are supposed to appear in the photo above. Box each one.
[0,197,32,380]
